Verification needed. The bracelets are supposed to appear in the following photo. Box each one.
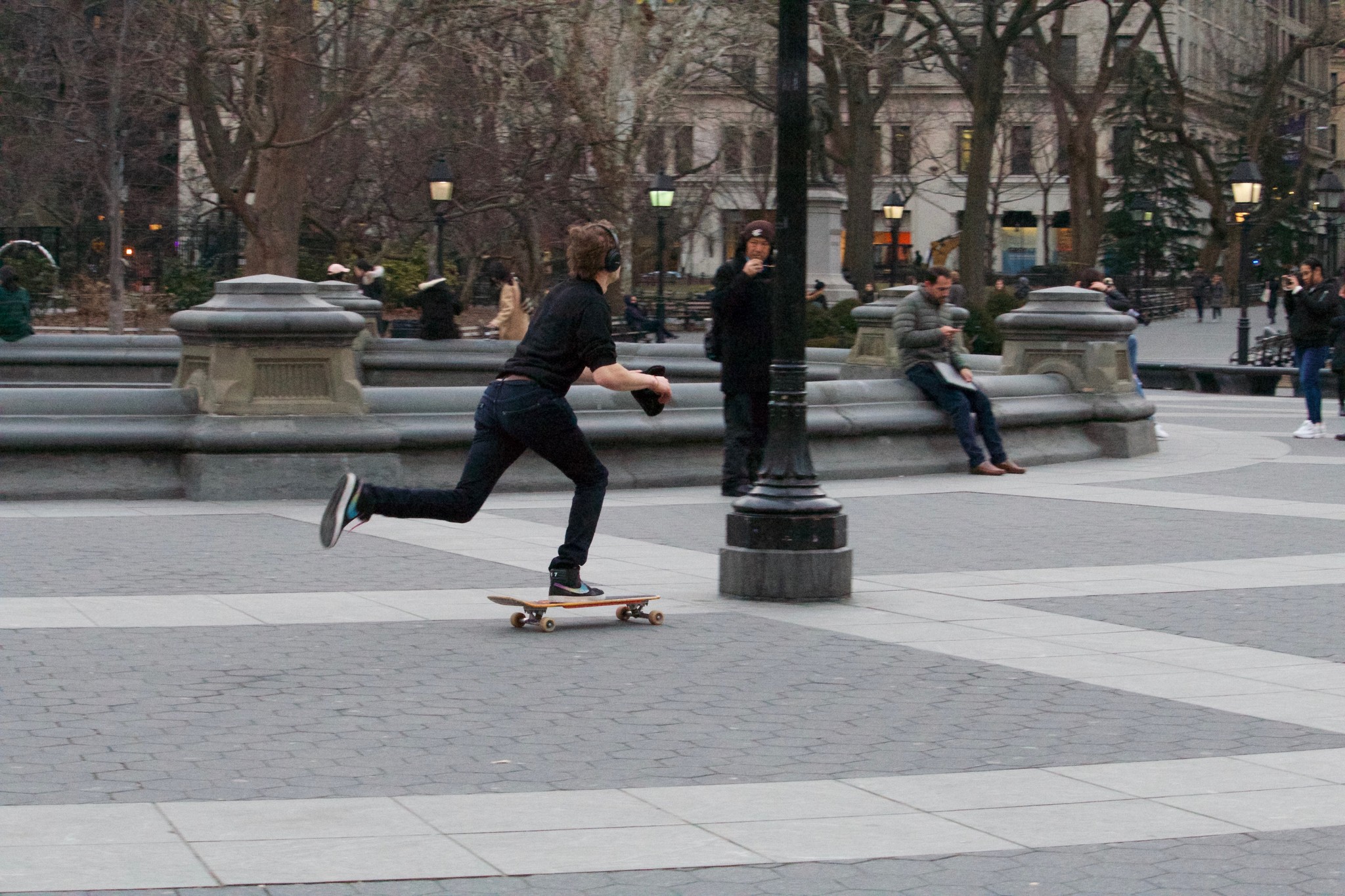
[652,375,658,391]
[1104,285,1109,292]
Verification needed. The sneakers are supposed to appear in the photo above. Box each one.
[320,473,370,549]
[1293,420,1327,439]
[549,570,606,603]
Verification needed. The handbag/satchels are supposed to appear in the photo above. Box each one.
[1261,281,1271,303]
[514,275,535,316]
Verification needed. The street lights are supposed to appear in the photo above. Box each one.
[1229,160,1263,364]
[881,190,905,288]
[427,176,453,280]
[644,185,677,323]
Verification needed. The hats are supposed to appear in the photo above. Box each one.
[327,263,351,275]
[743,220,775,245]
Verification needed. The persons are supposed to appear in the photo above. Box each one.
[478,262,530,341]
[1014,277,1034,299]
[945,271,966,307]
[893,265,1025,475]
[320,219,672,603]
[711,221,768,497]
[327,264,351,281]
[806,279,828,310]
[913,250,922,265]
[1074,269,1171,441]
[985,278,1024,318]
[624,295,680,343]
[0,265,35,341]
[1206,274,1224,322]
[416,274,462,340]
[1187,267,1210,323]
[859,282,880,303]
[1261,276,1280,324]
[353,259,384,300]
[1281,258,1345,442]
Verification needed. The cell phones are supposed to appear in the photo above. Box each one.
[954,325,965,329]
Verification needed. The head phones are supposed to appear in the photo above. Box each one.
[586,223,621,273]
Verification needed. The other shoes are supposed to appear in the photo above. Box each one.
[1155,421,1169,441]
[1336,433,1345,441]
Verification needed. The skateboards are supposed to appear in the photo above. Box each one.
[487,594,664,632]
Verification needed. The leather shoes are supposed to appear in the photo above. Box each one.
[973,462,1006,475]
[994,459,1025,474]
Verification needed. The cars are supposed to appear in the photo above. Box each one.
[641,271,682,279]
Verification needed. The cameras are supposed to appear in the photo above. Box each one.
[1286,273,1304,286]
